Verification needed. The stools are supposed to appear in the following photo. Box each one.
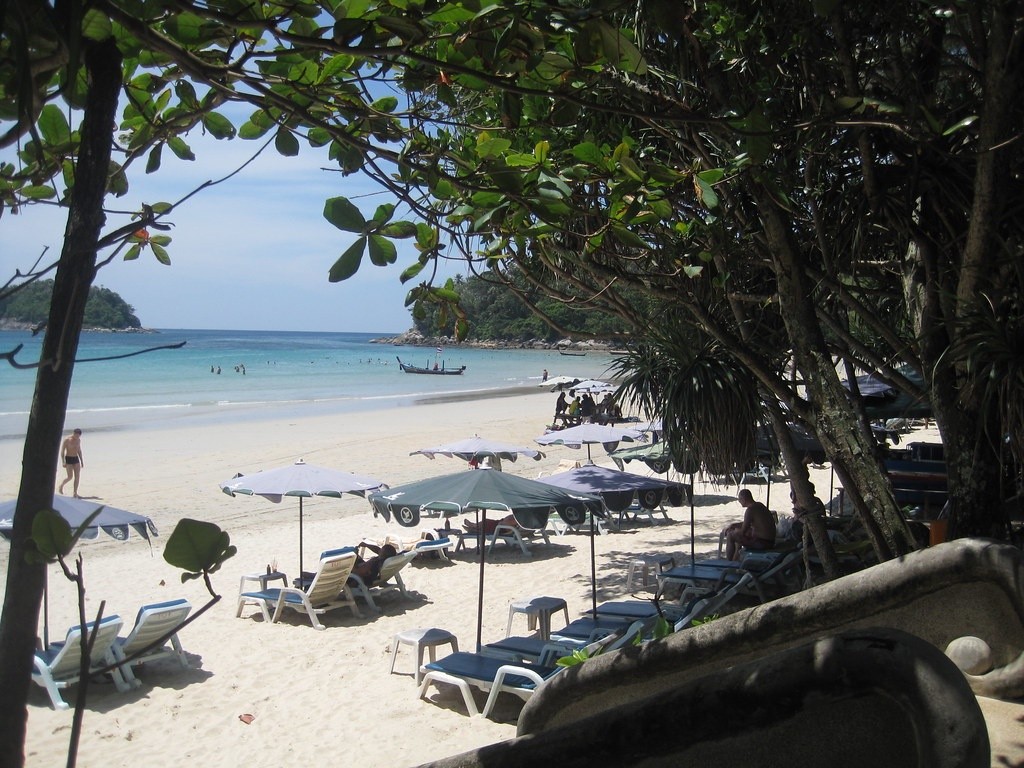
[626,554,675,593]
[437,528,466,555]
[390,628,460,688]
[504,596,570,641]
[237,571,289,607]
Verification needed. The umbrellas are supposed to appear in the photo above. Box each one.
[216,415,698,657]
[720,363,922,513]
[568,380,615,407]
[0,494,159,652]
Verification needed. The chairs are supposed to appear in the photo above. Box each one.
[362,528,454,568]
[417,419,951,720]
[235,546,367,631]
[294,549,423,611]
[49,598,193,692]
[31,615,132,711]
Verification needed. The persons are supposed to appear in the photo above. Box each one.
[217,366,221,374]
[59,429,83,498]
[725,489,776,561]
[554,392,568,423]
[346,542,396,588]
[569,396,582,423]
[605,393,614,411]
[581,394,593,417]
[542,369,548,382]
[240,364,245,374]
[809,482,826,516]
[462,514,518,534]
[599,395,608,406]
[211,366,214,373]
[235,366,239,372]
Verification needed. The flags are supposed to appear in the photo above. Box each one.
[436,347,442,352]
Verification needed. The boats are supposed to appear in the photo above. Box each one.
[396,356,464,374]
[559,349,588,356]
[395,343,403,346]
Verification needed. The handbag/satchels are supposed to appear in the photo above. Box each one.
[776,514,793,538]
